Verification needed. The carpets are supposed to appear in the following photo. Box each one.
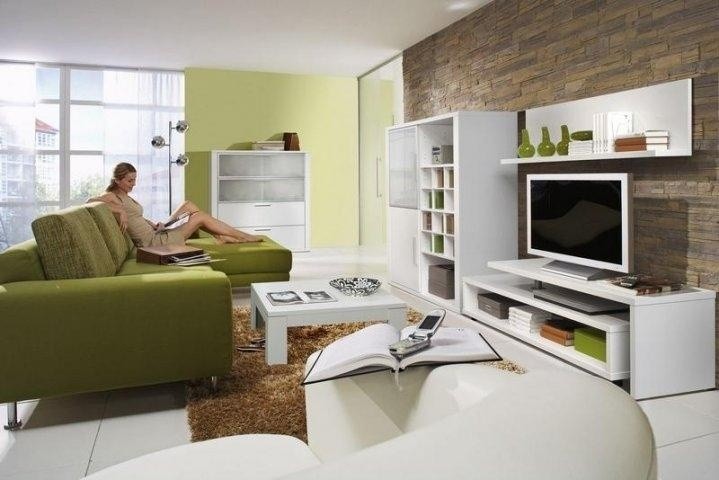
[186,305,527,445]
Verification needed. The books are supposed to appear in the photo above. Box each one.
[158,212,193,231]
[568,109,671,156]
[417,143,455,254]
[478,292,606,363]
[301,322,504,387]
[265,290,341,306]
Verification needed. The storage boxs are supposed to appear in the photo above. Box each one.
[428,264,454,299]
[477,293,521,319]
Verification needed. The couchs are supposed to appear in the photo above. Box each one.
[0,201,292,429]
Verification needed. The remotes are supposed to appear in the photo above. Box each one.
[620,276,640,288]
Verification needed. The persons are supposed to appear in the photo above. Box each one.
[85,161,266,249]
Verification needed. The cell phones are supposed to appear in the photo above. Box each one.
[388,308,447,360]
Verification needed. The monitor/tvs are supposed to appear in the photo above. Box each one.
[526,172,635,281]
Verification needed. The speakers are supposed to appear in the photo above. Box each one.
[432,144,453,164]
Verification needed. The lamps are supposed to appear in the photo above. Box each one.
[151,120,190,216]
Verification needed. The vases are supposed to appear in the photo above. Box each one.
[518,129,535,158]
[556,125,573,155]
[537,127,555,156]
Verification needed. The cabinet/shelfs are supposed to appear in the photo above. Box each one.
[461,257,715,400]
[186,150,311,253]
[384,109,518,315]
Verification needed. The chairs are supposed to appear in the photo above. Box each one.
[80,350,658,480]
